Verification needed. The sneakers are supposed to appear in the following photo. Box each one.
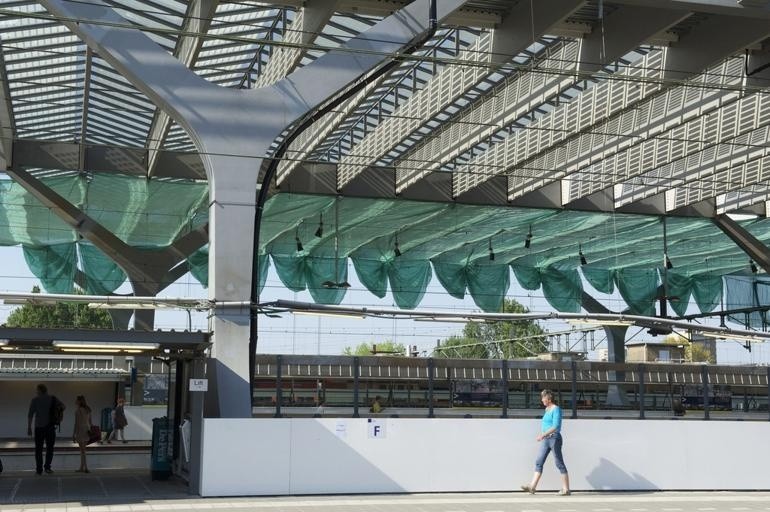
[107,440,112,443]
[122,441,129,443]
[521,486,536,495]
[36,468,53,475]
[558,489,570,495]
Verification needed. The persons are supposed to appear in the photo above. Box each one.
[27,385,66,475]
[99,399,129,444]
[373,395,385,413]
[72,396,95,473]
[521,389,570,496]
[318,401,326,417]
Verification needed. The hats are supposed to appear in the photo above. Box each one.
[118,399,126,402]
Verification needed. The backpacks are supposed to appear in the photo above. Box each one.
[50,395,63,425]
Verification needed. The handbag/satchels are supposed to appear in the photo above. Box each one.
[87,426,101,445]
